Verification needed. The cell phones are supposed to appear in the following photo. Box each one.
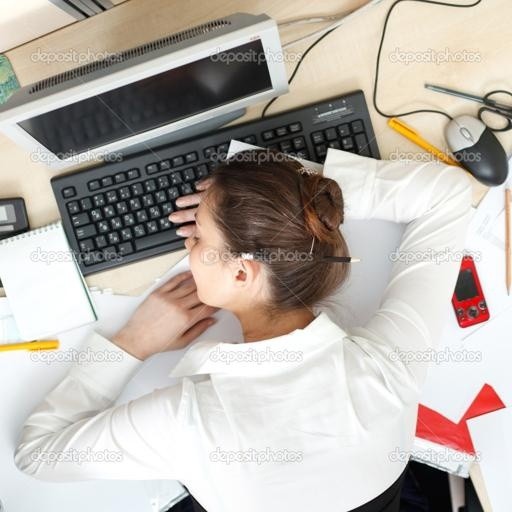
[451,255,490,328]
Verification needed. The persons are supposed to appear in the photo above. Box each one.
[14,148,472,512]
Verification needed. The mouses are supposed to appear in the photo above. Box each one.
[444,114,509,188]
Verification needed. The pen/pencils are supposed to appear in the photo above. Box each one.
[0,340,59,352]
[425,84,512,112]
[240,252,362,262]
[388,117,464,168]
[505,188,511,296]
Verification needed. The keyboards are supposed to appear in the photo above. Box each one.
[48,89,380,278]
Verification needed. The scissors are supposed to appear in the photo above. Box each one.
[478,90,512,131]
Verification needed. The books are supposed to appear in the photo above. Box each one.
[0,220,98,343]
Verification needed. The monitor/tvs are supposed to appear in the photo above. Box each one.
[0,11,291,170]
